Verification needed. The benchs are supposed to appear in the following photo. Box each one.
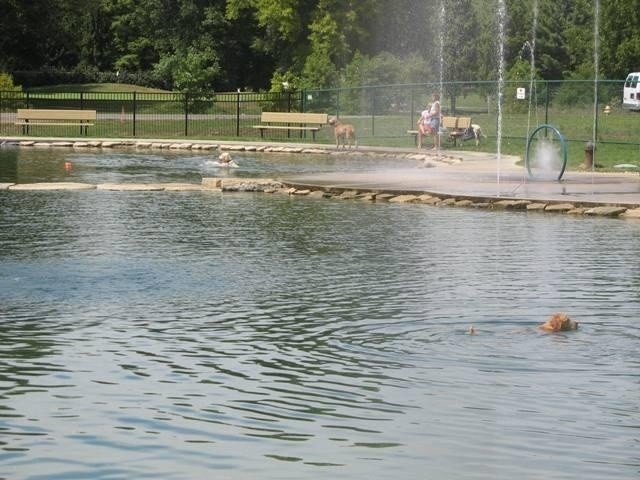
[253,112,328,143]
[407,117,472,149]
[15,109,96,136]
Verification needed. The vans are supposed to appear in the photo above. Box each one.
[622,72,640,110]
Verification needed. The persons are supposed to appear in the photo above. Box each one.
[426,92,441,150]
[416,102,433,148]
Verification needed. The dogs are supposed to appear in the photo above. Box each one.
[208,152,240,168]
[446,124,487,147]
[469,311,578,337]
[328,115,359,151]
